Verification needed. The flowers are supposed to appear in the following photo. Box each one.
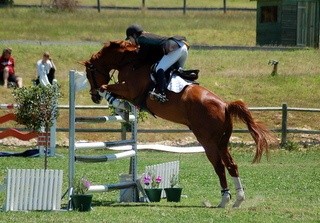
[143,174,162,189]
[75,178,91,195]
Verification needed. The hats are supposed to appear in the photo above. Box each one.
[124,24,143,41]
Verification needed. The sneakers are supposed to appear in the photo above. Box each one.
[148,90,167,104]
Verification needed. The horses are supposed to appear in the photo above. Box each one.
[83,39,280,208]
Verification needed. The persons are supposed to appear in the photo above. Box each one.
[0,47,22,89]
[125,25,199,104]
[36,52,56,88]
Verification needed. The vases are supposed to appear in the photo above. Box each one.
[70,194,93,212]
[144,189,163,203]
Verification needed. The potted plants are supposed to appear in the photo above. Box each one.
[164,174,182,203]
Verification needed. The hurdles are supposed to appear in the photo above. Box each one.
[61,69,149,210]
